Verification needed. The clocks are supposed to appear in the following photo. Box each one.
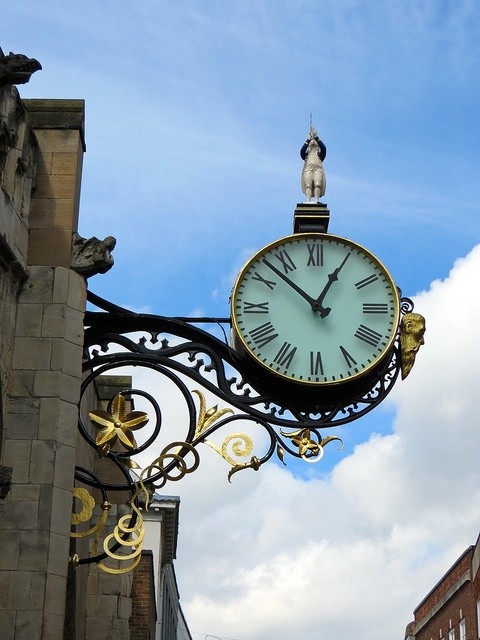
[231,228,401,389]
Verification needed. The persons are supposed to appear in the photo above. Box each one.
[300,128,326,204]
